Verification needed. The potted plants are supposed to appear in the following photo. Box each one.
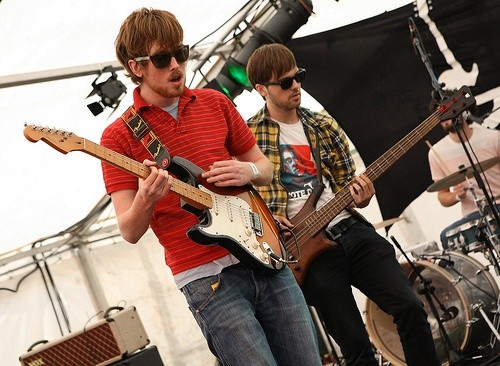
[203,68,245,108]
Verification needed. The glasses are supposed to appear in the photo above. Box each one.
[133,45,189,69]
[261,68,306,91]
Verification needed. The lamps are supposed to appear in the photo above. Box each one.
[85,65,127,116]
[221,0,317,95]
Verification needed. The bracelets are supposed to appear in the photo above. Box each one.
[249,162,260,180]
[456,194,465,201]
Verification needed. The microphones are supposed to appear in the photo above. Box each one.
[408,18,420,55]
[440,307,459,322]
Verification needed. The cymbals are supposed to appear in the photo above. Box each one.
[426,156,500,193]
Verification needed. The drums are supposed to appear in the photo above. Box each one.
[439,203,500,255]
[365,251,500,366]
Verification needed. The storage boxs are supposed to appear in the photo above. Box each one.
[19,303,150,366]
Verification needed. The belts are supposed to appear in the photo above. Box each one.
[326,215,358,240]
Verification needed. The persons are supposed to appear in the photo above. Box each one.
[101,7,323,366]
[243,44,442,365]
[428,90,500,275]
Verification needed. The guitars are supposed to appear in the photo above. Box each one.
[24,125,287,271]
[278,86,476,284]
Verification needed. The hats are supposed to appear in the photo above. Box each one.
[430,88,444,113]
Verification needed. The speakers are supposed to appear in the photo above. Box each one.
[18,306,150,366]
